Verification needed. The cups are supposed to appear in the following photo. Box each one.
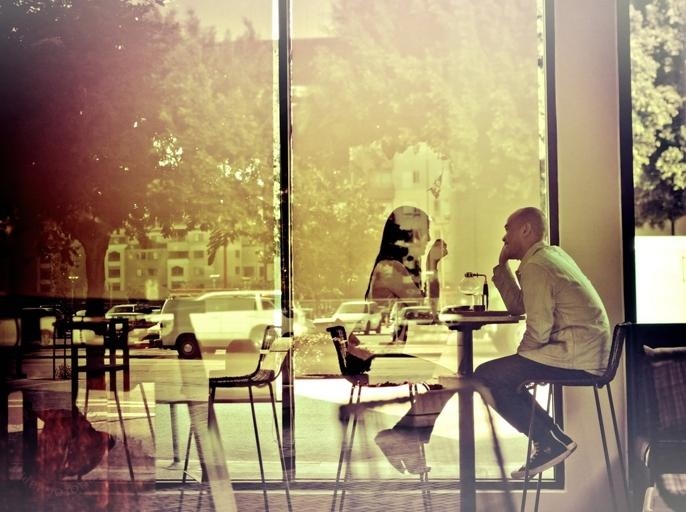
[472,294,486,312]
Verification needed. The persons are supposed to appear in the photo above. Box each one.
[472,206,610,478]
[344,204,457,475]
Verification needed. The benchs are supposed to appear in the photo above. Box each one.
[621,321,686,512]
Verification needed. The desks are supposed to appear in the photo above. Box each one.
[404,309,522,512]
[153,380,285,512]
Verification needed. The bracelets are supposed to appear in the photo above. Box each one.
[426,269,438,281]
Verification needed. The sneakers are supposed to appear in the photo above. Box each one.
[509,429,571,480]
[552,424,578,455]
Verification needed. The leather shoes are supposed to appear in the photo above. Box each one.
[373,429,406,475]
[402,451,432,476]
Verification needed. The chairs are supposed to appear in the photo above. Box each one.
[327,322,454,512]
[1,310,157,512]
[177,325,298,512]
[513,319,634,512]
